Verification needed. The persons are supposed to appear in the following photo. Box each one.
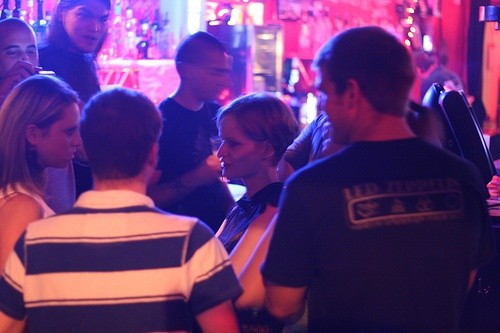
[259,23,488,333]
[276,107,345,185]
[37,0,112,199]
[411,50,464,107]
[0,87,244,333]
[212,92,302,333]
[0,18,79,216]
[1,76,82,280]
[145,31,236,236]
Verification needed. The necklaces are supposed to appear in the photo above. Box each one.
[245,178,281,195]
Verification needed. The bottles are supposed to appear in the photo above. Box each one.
[31,0,48,44]
[111,1,122,57]
[122,10,136,60]
[150,8,162,45]
[1,0,10,20]
[12,0,21,18]
